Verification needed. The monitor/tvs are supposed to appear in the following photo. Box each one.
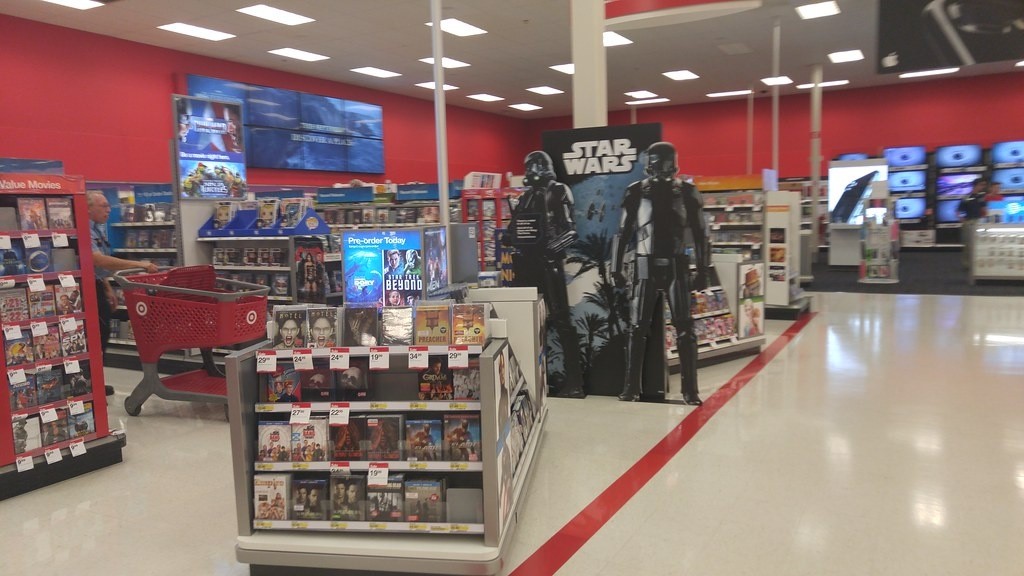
[837,141,1024,227]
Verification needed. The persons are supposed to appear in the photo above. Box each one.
[384,249,422,306]
[310,314,334,347]
[957,178,987,222]
[87,189,158,396]
[274,316,301,349]
[304,252,324,293]
[257,360,478,522]
[498,352,508,439]
[982,182,1003,216]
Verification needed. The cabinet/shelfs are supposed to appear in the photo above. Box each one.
[180,199,479,374]
[0,173,123,501]
[663,190,766,367]
[226,287,550,576]
[766,173,829,320]
[102,200,182,376]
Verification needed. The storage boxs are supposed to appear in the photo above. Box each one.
[212,173,528,297]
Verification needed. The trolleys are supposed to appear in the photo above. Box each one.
[110,264,271,422]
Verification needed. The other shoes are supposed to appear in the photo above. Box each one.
[105,385,114,395]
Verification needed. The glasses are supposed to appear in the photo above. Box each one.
[90,203,110,208]
